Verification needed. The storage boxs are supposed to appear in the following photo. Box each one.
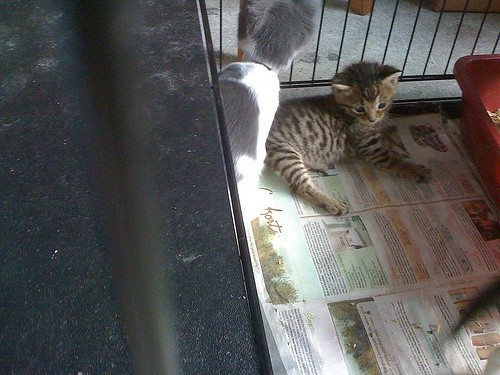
[454,53,500,209]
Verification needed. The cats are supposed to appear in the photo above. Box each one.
[265,61,434,215]
[217,0,315,220]
